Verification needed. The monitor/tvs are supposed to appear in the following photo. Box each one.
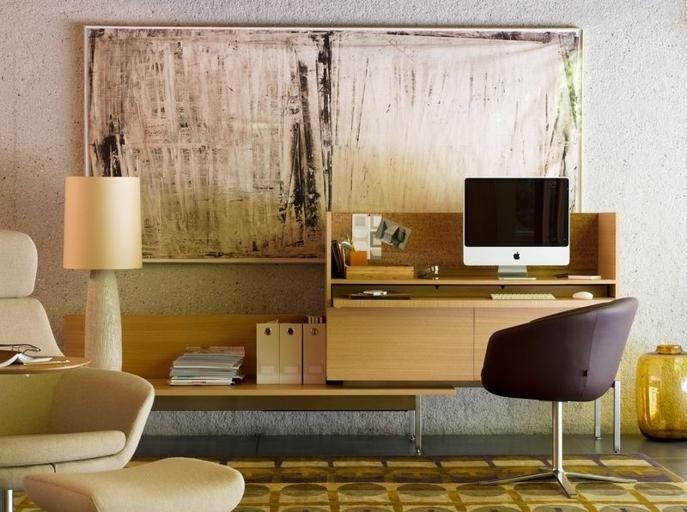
[463,177,570,280]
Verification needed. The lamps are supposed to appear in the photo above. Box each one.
[63,176,143,371]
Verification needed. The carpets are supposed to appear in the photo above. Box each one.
[14,451,687,512]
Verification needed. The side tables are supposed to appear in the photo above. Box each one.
[0,356,91,375]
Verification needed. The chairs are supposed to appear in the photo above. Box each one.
[0,230,155,511]
[455,297,639,500]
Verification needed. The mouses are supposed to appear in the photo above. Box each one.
[572,291,593,299]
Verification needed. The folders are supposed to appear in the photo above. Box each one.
[255,319,327,385]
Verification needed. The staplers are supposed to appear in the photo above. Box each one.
[417,265,440,279]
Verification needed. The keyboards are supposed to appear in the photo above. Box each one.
[490,293,556,300]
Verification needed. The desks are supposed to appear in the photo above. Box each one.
[143,378,455,457]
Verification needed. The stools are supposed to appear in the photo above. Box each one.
[24,455,245,512]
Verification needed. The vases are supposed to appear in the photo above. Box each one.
[634,345,686,440]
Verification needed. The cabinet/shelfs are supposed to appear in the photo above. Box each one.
[327,210,620,455]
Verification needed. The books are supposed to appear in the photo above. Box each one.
[167,344,249,387]
[0,348,52,368]
[556,274,602,280]
